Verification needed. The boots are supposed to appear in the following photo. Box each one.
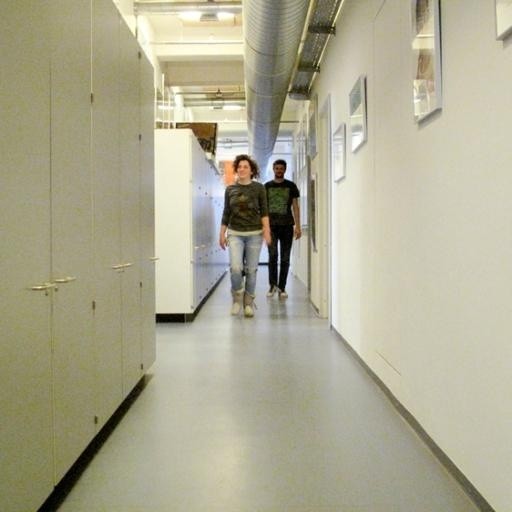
[230,286,245,316]
[244,289,256,317]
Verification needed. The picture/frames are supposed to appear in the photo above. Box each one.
[330,1,512,184]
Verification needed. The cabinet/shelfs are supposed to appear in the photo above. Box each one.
[0,2,157,511]
[154,129,228,322]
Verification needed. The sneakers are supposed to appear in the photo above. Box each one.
[265,284,289,298]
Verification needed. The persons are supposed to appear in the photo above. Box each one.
[219,153,273,317]
[263,158,302,299]
